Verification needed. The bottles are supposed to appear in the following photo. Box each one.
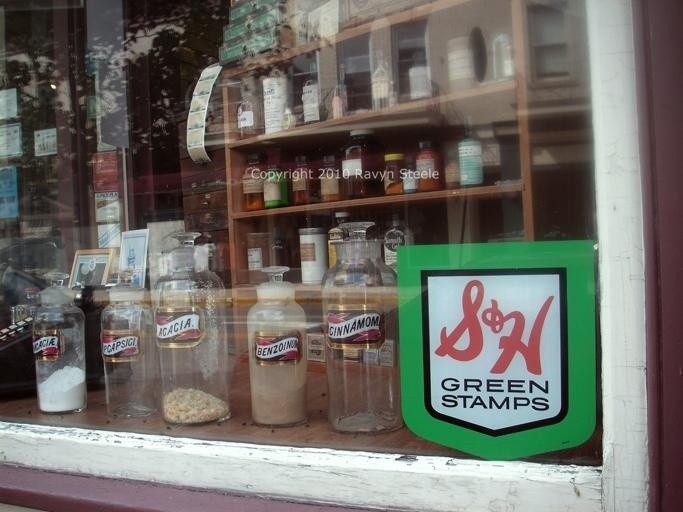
[236,125,506,212]
[442,18,477,94]
[240,263,310,430]
[331,62,352,120]
[263,75,295,134]
[28,269,87,416]
[298,208,417,285]
[298,58,320,128]
[246,233,271,284]
[319,221,403,435]
[149,232,231,424]
[368,53,393,110]
[100,263,155,421]
[232,76,262,143]
[406,38,433,103]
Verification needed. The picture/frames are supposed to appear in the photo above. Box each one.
[67,249,116,288]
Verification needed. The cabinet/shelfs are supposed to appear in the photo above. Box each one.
[222,4,595,304]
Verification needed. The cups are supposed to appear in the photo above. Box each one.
[486,26,514,81]
